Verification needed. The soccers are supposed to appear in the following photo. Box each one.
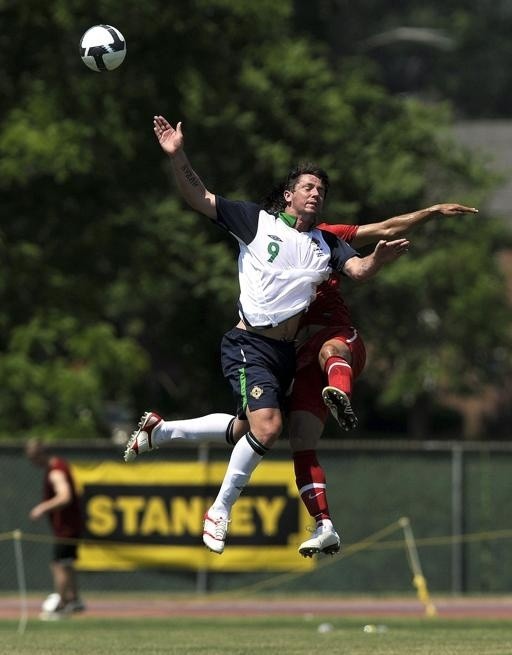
[80,23,126,69]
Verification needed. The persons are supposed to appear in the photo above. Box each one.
[260,198,479,558]
[123,113,411,555]
[24,435,89,615]
